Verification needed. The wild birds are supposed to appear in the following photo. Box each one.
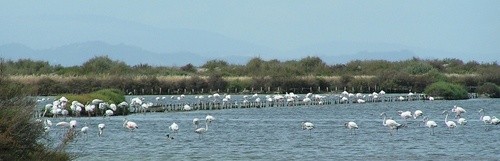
[192,114,217,140]
[183,103,191,111]
[194,93,232,106]
[339,90,386,105]
[121,117,139,134]
[176,94,187,103]
[81,126,89,141]
[170,95,175,100]
[41,95,69,119]
[242,93,261,104]
[379,109,438,136]
[97,123,106,140]
[35,118,53,136]
[302,92,324,107]
[166,134,174,140]
[69,100,84,119]
[301,121,315,136]
[398,95,404,100]
[441,104,469,136]
[408,89,414,96]
[168,121,180,134]
[427,95,435,101]
[117,101,128,116]
[265,91,300,108]
[155,96,166,105]
[85,98,117,120]
[478,108,500,133]
[344,121,359,136]
[55,119,78,136]
[128,91,154,115]
